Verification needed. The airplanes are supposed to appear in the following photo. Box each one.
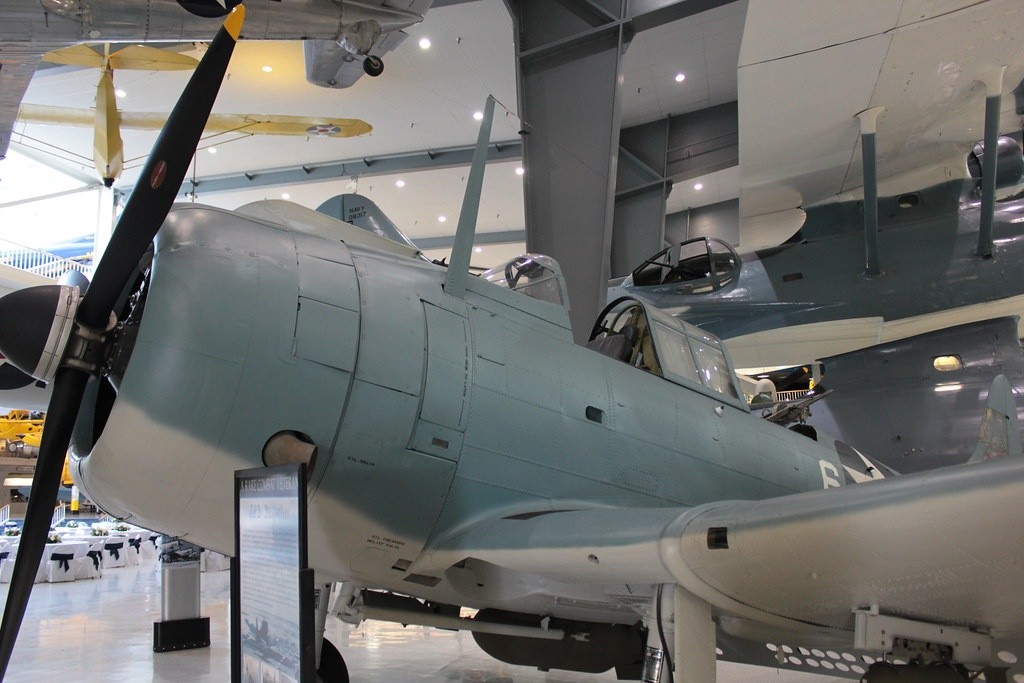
[0,0,1024,683]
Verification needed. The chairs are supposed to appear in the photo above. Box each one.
[0,521,231,583]
